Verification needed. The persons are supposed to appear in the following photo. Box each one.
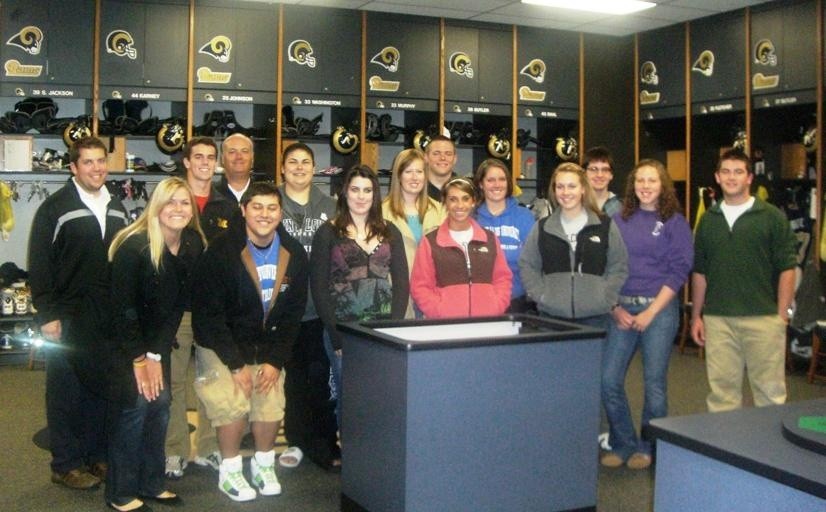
[381,149,448,319]
[163,136,234,479]
[584,145,627,450]
[410,178,513,318]
[277,144,341,476]
[28,136,136,489]
[309,165,410,447]
[213,131,262,207]
[422,135,463,199]
[189,187,308,502]
[595,161,696,470]
[468,159,541,314]
[518,162,629,361]
[690,150,799,414]
[98,176,209,511]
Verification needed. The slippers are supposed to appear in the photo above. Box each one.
[281,445,303,469]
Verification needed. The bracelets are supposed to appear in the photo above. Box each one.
[145,351,162,362]
[134,357,148,368]
[230,368,241,373]
[611,303,619,310]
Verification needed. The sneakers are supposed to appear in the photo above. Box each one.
[599,432,653,470]
[0,290,29,316]
[50,452,283,512]
[0,321,34,350]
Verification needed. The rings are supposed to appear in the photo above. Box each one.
[258,370,263,375]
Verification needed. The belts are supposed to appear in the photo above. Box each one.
[618,295,656,304]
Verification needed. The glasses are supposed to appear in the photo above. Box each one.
[586,165,613,173]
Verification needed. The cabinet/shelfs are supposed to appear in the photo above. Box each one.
[0,89,96,185]
[273,98,363,185]
[430,107,515,186]
[0,314,37,358]
[185,98,279,180]
[89,96,188,183]
[628,106,824,380]
[511,110,586,191]
[640,398,825,512]
[361,105,439,184]
